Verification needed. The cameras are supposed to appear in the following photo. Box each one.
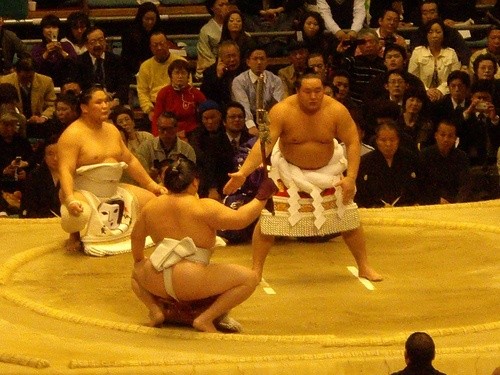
[51,39,62,54]
[341,36,366,46]
[9,156,22,170]
[475,101,489,110]
[383,34,397,47]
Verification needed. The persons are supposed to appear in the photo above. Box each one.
[224,66,384,282]
[55,84,170,256]
[391,332,446,375]
[132,157,281,333]
[0,0,500,218]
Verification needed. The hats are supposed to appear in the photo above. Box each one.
[198,101,225,114]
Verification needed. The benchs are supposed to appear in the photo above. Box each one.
[0,0,500,65]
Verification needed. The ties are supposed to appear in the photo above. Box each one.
[95,57,104,83]
[231,138,238,153]
[456,104,461,116]
[478,115,487,144]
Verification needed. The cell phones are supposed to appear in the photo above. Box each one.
[218,57,228,72]
[154,159,159,169]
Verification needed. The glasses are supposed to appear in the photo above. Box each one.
[229,115,245,119]
[388,80,405,85]
